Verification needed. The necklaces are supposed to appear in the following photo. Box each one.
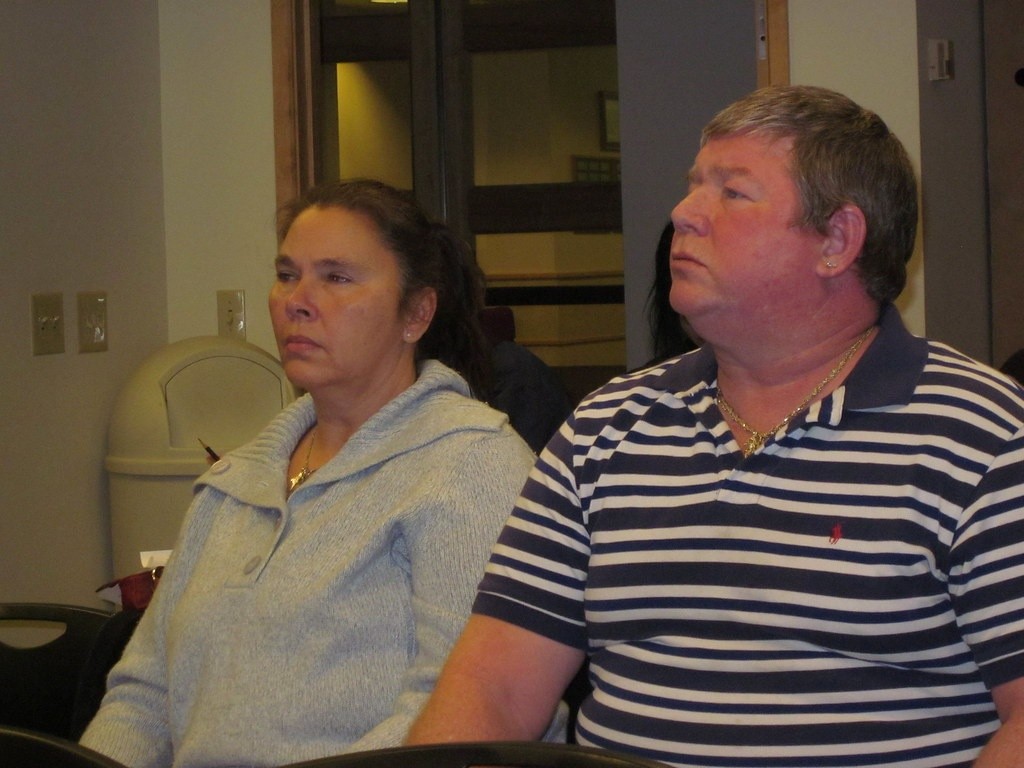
[290,426,319,494]
[715,326,873,457]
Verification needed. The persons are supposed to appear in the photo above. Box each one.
[79,178,569,768]
[404,84,1024,768]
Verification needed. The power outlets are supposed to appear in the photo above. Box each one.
[215,289,247,341]
[76,290,110,355]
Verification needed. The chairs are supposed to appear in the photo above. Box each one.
[0,602,114,740]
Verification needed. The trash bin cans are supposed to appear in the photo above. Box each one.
[106,337,303,590]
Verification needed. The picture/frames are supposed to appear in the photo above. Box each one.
[598,93,623,154]
